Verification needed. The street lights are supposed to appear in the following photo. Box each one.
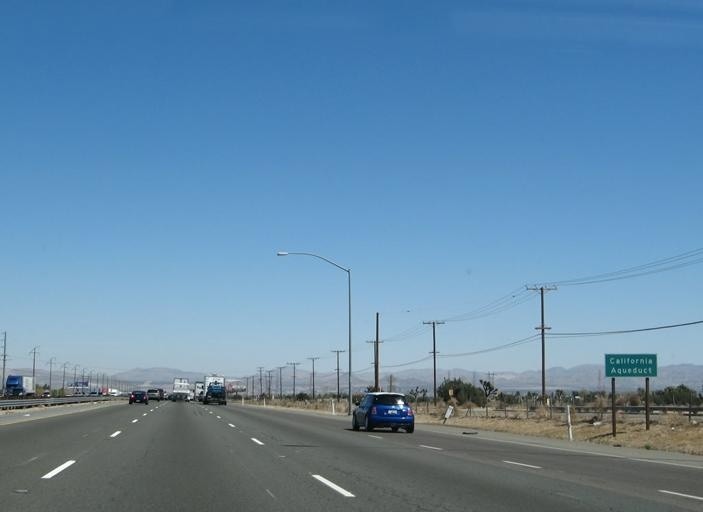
[276,249,353,418]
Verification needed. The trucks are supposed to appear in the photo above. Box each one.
[3,375,34,397]
[66,382,132,396]
[172,375,228,405]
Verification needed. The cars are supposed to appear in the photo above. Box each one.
[27,392,38,397]
[42,390,53,398]
[348,389,418,435]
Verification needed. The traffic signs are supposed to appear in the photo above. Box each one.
[604,353,658,377]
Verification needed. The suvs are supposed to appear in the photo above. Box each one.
[10,387,26,397]
[128,388,173,405]
